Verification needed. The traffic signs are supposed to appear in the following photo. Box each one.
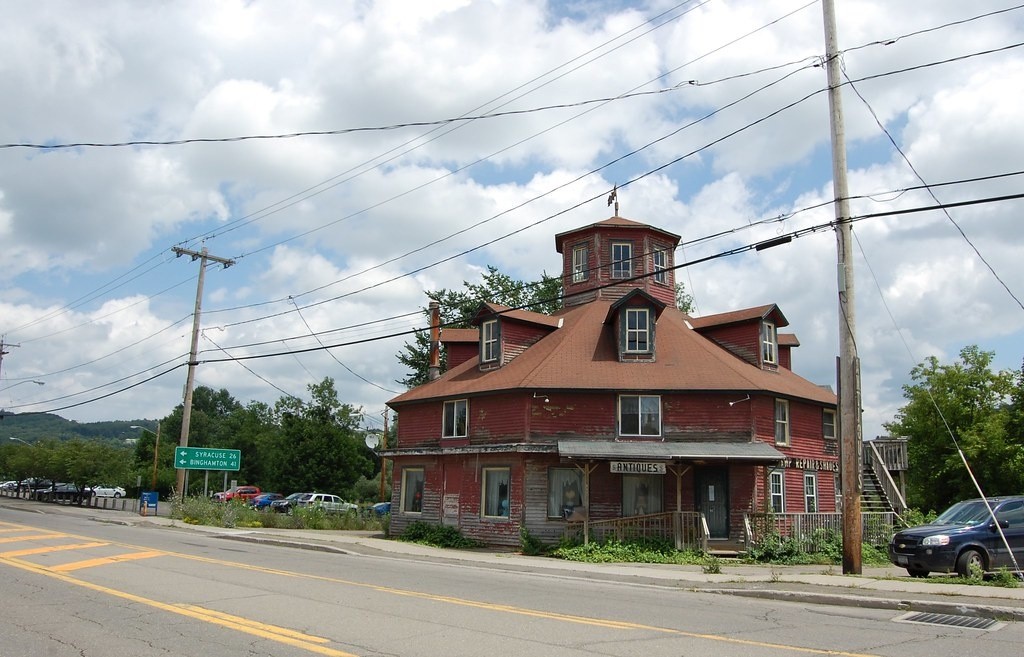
[174,446,241,471]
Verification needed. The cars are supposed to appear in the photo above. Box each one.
[366,502,391,519]
[271,492,309,515]
[297,492,359,520]
[91,484,127,499]
[0,476,92,503]
[246,492,285,511]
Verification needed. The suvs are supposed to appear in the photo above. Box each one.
[888,495,1024,580]
[212,486,261,504]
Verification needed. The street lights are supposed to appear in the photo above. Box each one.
[130,422,161,491]
